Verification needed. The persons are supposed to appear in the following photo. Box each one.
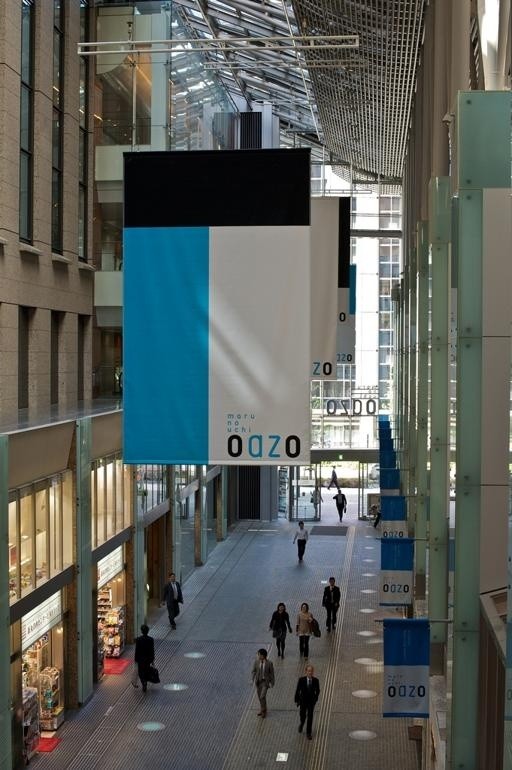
[309,486,324,518]
[333,489,347,522]
[251,648,276,719]
[322,577,341,632]
[294,665,320,739]
[328,466,340,490]
[373,497,381,527]
[293,520,310,562]
[161,571,184,628]
[267,602,292,658]
[296,602,314,661]
[134,625,155,691]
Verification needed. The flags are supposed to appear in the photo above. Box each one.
[311,196,350,380]
[124,146,313,465]
[338,263,356,366]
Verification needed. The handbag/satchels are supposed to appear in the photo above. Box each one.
[131,663,139,689]
[148,664,161,684]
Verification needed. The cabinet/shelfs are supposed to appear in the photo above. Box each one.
[22,642,65,761]
[97,586,126,683]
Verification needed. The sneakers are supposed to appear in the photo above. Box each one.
[277,650,310,662]
[297,724,314,741]
[256,710,267,719]
[324,624,337,632]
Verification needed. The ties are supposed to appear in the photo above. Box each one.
[173,583,177,600]
[308,679,312,693]
[260,661,265,681]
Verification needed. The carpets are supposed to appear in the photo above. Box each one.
[102,657,133,675]
